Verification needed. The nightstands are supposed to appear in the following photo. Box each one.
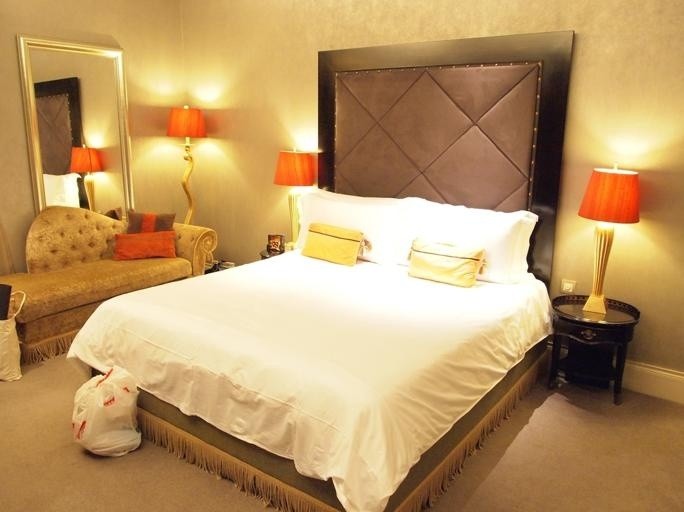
[258,246,284,259]
[551,293,639,406]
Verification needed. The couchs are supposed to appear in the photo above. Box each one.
[0,204,218,382]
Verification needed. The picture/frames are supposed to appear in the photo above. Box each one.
[267,234,282,250]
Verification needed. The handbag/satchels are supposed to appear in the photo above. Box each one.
[71,364,142,459]
[0,291,31,384]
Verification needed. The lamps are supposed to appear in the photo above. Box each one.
[67,142,107,211]
[272,144,319,247]
[162,102,207,228]
[576,162,643,318]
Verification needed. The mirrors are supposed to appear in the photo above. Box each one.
[14,33,135,223]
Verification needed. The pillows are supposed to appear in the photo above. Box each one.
[287,182,400,272]
[394,194,539,289]
[125,208,176,235]
[103,206,123,220]
[302,222,368,270]
[111,229,177,260]
[407,236,488,289]
[43,172,81,207]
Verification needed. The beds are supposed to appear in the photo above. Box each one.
[66,28,580,512]
[31,76,88,212]
